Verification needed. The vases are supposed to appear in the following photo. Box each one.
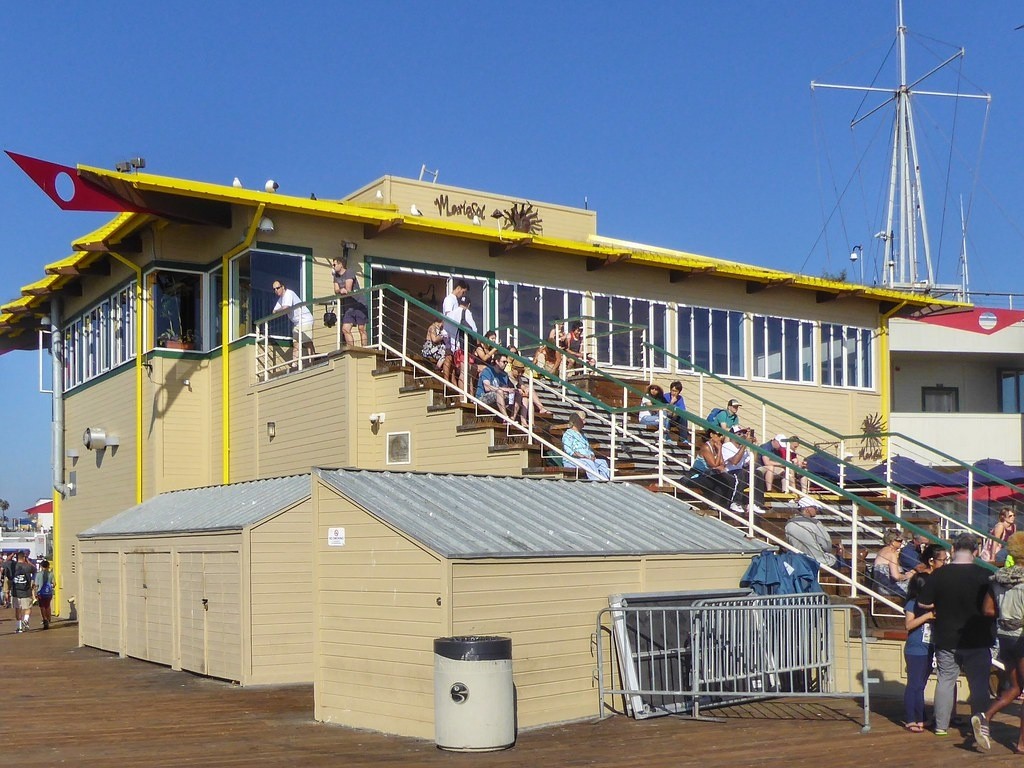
[165,340,195,349]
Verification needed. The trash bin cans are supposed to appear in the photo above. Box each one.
[433,635,516,752]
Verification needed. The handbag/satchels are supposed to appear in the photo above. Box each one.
[37,583,52,599]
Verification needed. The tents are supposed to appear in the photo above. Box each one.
[740,549,823,692]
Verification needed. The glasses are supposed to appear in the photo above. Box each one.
[273,285,282,291]
[332,264,340,267]
[651,386,659,390]
[714,432,723,437]
[1005,514,1015,518]
[935,556,950,562]
[499,361,508,364]
[893,539,903,543]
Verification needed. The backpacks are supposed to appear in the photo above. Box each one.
[454,309,477,353]
[707,407,727,423]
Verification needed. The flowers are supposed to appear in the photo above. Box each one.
[158,276,195,345]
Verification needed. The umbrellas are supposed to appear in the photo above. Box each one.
[806,453,1024,525]
[23,502,53,513]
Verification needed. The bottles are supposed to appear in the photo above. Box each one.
[836,542,844,557]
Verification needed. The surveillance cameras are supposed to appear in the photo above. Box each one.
[265,180,280,193]
[369,414,377,424]
[850,253,857,261]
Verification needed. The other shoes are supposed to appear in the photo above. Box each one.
[765,491,796,504]
[502,409,554,429]
[837,543,870,563]
[16,616,50,633]
[904,710,1024,755]
[4,602,11,608]
[32,599,37,605]
[655,436,693,446]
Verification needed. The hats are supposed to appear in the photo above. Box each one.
[797,497,822,509]
[512,359,524,371]
[459,296,471,305]
[727,400,742,406]
[775,434,786,447]
[729,426,747,435]
[647,381,664,393]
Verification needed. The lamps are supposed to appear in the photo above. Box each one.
[131,157,146,176]
[342,240,357,251]
[267,421,275,437]
[115,162,130,174]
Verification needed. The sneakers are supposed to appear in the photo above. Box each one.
[747,504,766,514]
[729,503,745,513]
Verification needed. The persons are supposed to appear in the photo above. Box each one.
[0,550,56,633]
[474,331,554,427]
[904,533,1024,753]
[873,529,929,595]
[562,411,609,480]
[684,424,766,513]
[423,319,447,371]
[981,507,1017,562]
[756,434,807,493]
[784,496,868,570]
[534,320,596,383]
[333,256,369,346]
[440,280,477,405]
[706,399,742,439]
[639,381,688,443]
[273,279,315,368]
